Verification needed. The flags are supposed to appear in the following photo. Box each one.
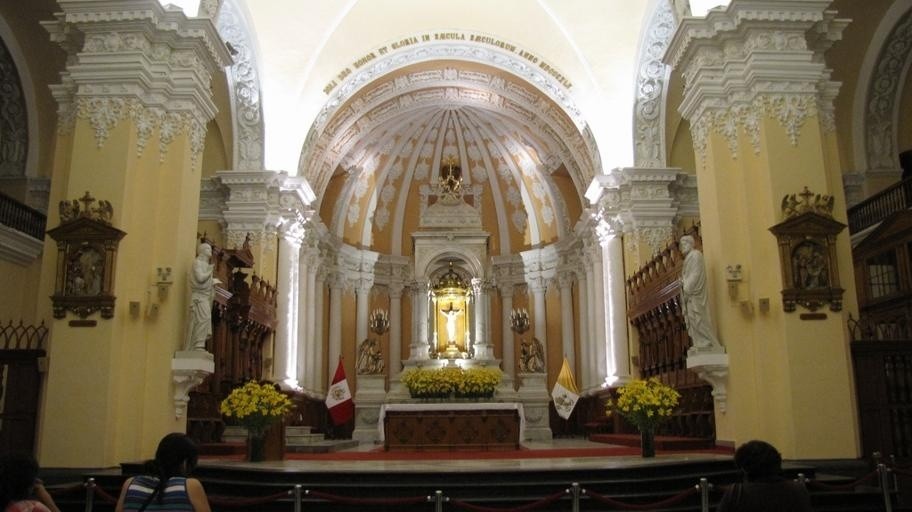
[549,357,581,420]
[325,357,353,426]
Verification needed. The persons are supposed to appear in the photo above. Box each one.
[358,341,383,374]
[440,309,463,341]
[0,450,60,512]
[114,432,212,512]
[183,242,215,351]
[519,337,544,372]
[679,235,720,347]
[716,441,813,512]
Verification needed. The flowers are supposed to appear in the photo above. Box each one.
[604,375,684,431]
[401,365,504,396]
[220,377,291,426]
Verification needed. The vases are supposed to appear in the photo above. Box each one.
[641,430,655,457]
[249,425,266,462]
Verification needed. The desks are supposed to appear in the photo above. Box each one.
[384,402,521,451]
